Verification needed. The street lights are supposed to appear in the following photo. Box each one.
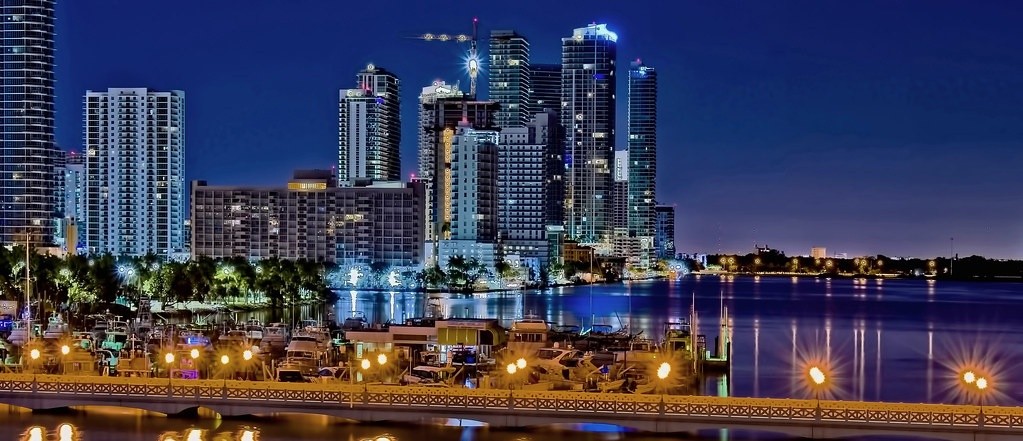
[507,363,517,412]
[811,367,826,401]
[31,349,40,392]
[165,353,174,398]
[657,361,671,395]
[61,345,70,375]
[964,371,987,406]
[378,354,387,383]
[517,357,527,390]
[221,355,229,399]
[362,360,370,403]
[191,349,198,370]
[244,350,252,380]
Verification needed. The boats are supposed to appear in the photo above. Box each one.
[0,295,729,395]
